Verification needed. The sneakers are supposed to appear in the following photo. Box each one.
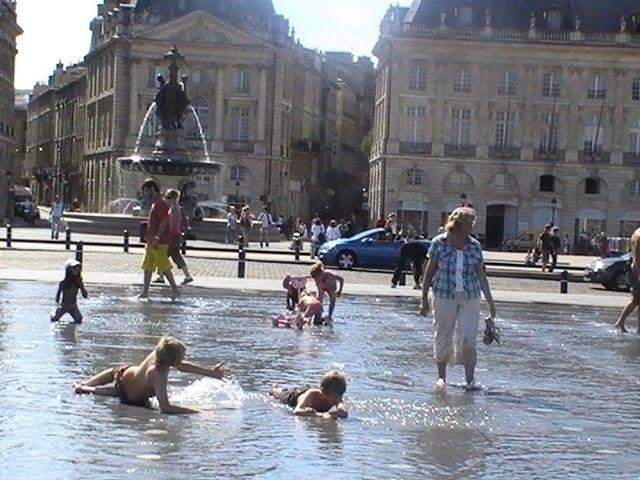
[391,282,396,288]
[180,278,193,285]
[152,278,165,283]
[413,284,422,290]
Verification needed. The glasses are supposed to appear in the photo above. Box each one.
[165,196,173,199]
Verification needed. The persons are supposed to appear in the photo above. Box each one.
[337,218,347,238]
[283,277,305,311]
[277,215,286,235]
[50,260,87,324]
[298,291,330,325]
[590,234,599,256]
[312,212,323,224]
[258,206,273,248]
[73,336,231,415]
[550,227,561,271]
[393,240,434,290]
[437,224,445,235]
[311,219,324,256]
[562,234,570,255]
[385,213,403,242]
[326,220,342,244]
[598,231,610,259]
[578,228,586,255]
[549,222,555,233]
[417,207,497,390]
[239,206,252,249]
[135,178,181,302]
[310,265,343,321]
[225,205,238,244]
[296,216,300,227]
[340,221,354,239]
[49,195,62,240]
[615,226,640,334]
[289,220,305,251]
[537,224,556,273]
[587,233,592,256]
[153,189,193,287]
[287,215,294,241]
[267,372,349,421]
[376,215,385,240]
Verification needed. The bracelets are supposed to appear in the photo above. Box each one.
[155,236,159,240]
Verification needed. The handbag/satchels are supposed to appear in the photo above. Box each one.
[241,218,250,228]
[318,233,326,242]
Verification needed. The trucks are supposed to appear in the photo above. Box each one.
[504,231,540,251]
[8,186,40,218]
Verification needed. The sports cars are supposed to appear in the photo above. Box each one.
[317,226,432,274]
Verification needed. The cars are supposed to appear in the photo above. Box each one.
[586,252,634,292]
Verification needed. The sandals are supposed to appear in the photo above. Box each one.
[482,314,494,345]
[484,317,503,346]
